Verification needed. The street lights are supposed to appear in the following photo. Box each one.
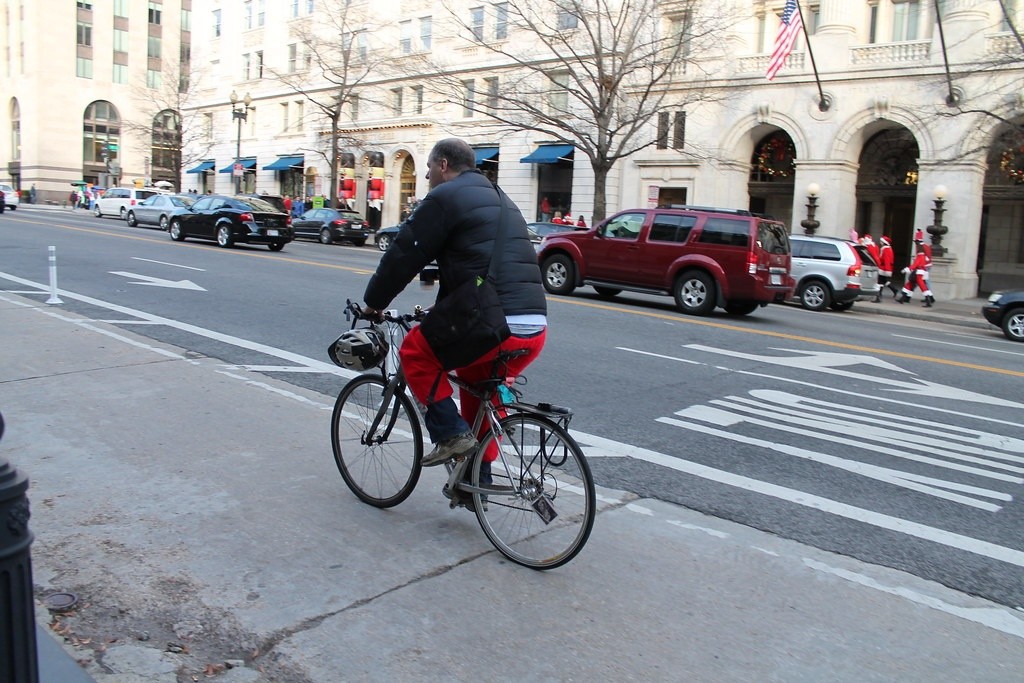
[230,90,253,196]
[801,183,820,234]
[927,184,948,257]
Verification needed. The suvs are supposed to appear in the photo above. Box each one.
[788,233,881,312]
[536,204,796,317]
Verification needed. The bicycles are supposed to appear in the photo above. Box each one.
[331,299,596,570]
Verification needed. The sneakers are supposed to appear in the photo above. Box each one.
[420,430,480,467]
[442,486,488,511]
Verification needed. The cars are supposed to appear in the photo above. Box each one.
[125,195,202,231]
[249,195,288,214]
[374,214,413,251]
[0,184,21,214]
[526,222,591,253]
[164,194,295,251]
[93,188,159,220]
[292,208,369,246]
[981,288,1024,342]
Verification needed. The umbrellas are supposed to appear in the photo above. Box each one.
[154,181,174,188]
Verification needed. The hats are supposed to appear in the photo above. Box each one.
[880,236,892,245]
[914,232,923,242]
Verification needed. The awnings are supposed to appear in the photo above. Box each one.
[472,148,498,164]
[187,162,215,173]
[263,156,304,170]
[509,144,574,163]
[219,160,256,173]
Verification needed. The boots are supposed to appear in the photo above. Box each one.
[894,288,909,304]
[922,292,932,307]
[878,284,883,296]
[885,281,898,297]
[904,290,915,303]
[922,290,935,302]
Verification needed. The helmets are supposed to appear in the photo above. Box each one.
[328,324,389,371]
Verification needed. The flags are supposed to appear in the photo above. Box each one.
[765,0,804,82]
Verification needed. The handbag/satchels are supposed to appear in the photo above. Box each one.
[419,272,510,370]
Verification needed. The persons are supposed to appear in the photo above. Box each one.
[356,139,548,512]
[15,188,22,198]
[188,189,268,195]
[281,195,421,234]
[849,228,899,303]
[70,190,101,210]
[29,183,36,204]
[895,229,936,307]
[540,196,586,228]
